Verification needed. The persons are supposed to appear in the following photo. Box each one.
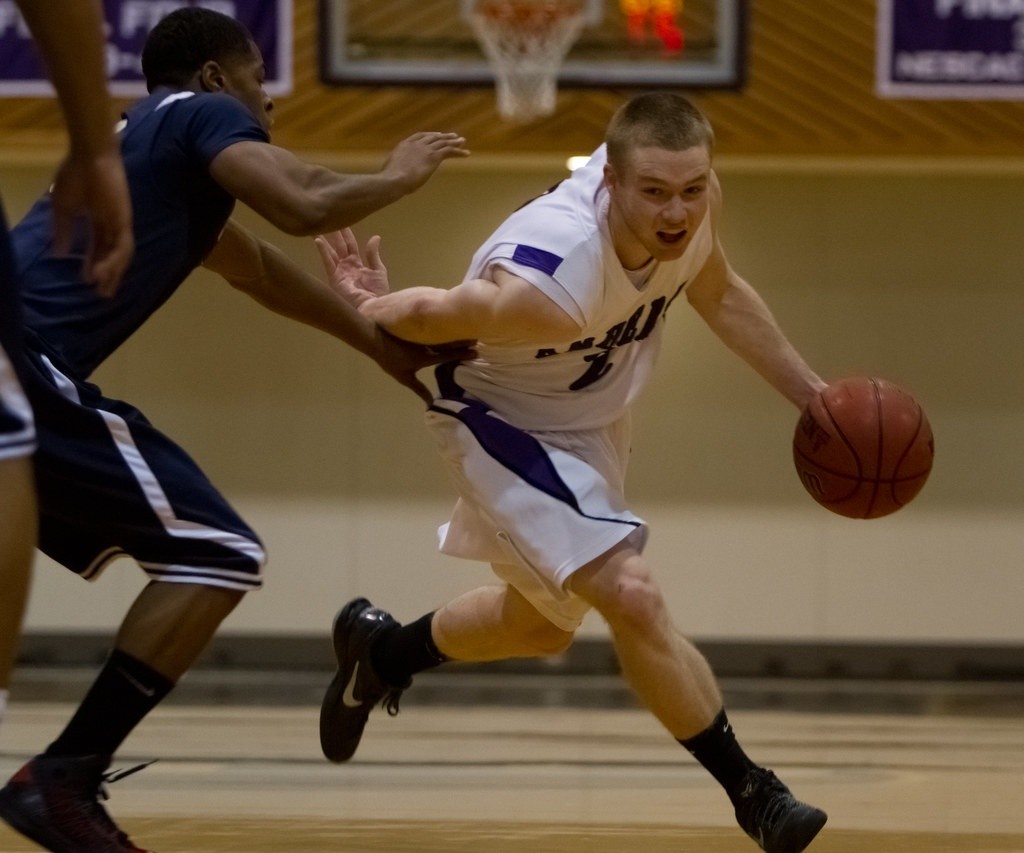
[314,90,827,853]
[0,8,471,853]
[0,0,137,701]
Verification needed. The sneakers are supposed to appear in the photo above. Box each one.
[0,754,160,853]
[735,770,828,853]
[320,598,412,762]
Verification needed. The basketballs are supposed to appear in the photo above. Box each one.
[793,374,934,522]
